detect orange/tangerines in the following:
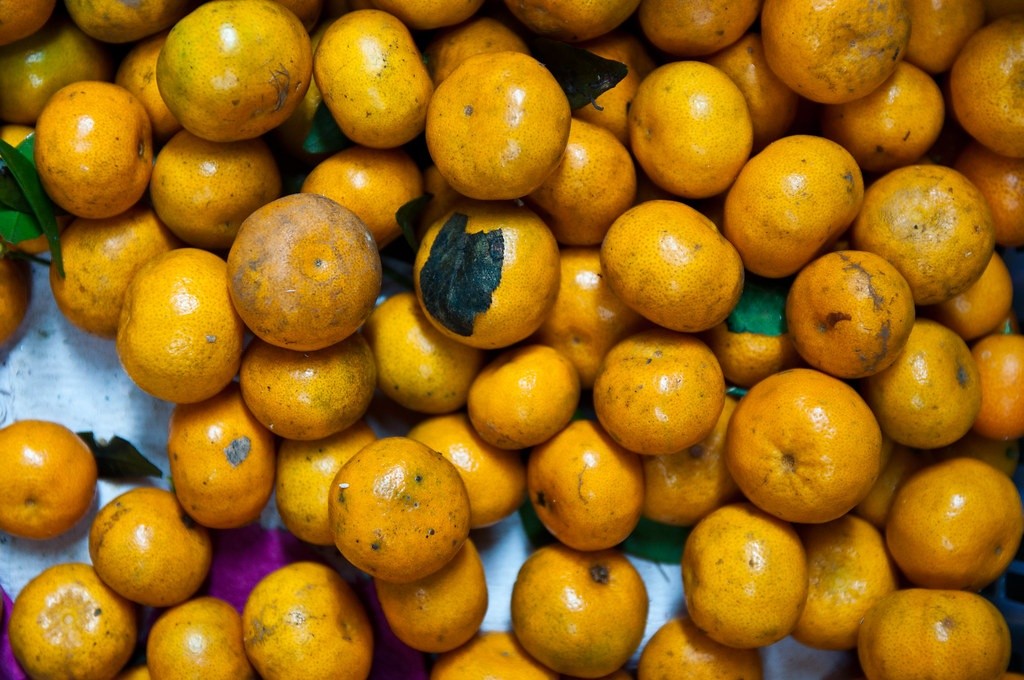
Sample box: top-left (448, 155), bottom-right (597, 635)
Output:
top-left (0, 0), bottom-right (1024, 680)
